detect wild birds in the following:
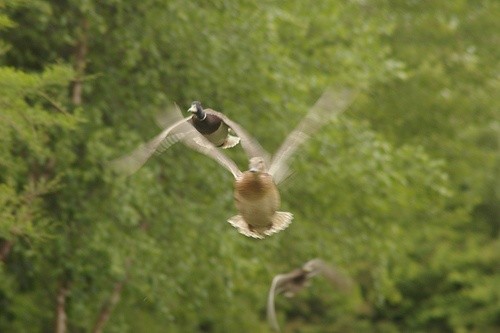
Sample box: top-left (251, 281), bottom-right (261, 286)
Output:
top-left (123, 99), bottom-right (288, 185)
top-left (148, 86), bottom-right (348, 239)
top-left (266, 255), bottom-right (352, 333)
